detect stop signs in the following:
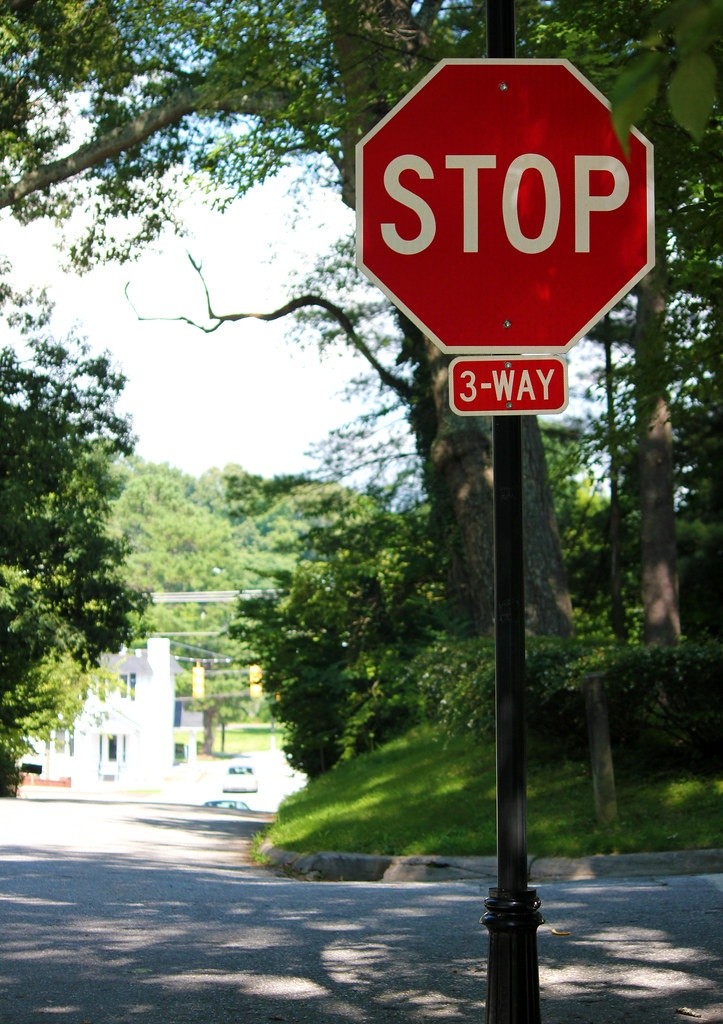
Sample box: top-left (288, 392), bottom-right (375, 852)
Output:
top-left (356, 57), bottom-right (656, 355)
